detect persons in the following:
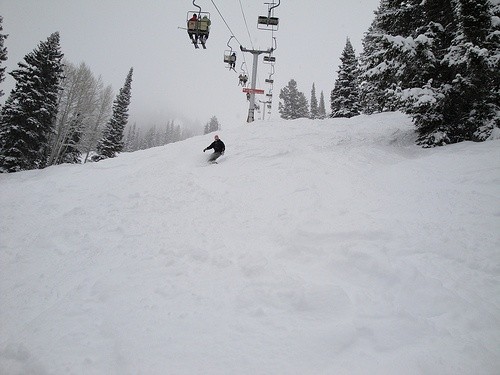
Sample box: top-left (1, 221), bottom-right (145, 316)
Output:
top-left (242, 75), bottom-right (248, 87)
top-left (230, 53), bottom-right (236, 69)
top-left (199, 15), bottom-right (211, 49)
top-left (187, 14), bottom-right (199, 48)
top-left (247, 93), bottom-right (250, 99)
top-left (238, 74), bottom-right (242, 85)
top-left (203, 135), bottom-right (226, 164)
